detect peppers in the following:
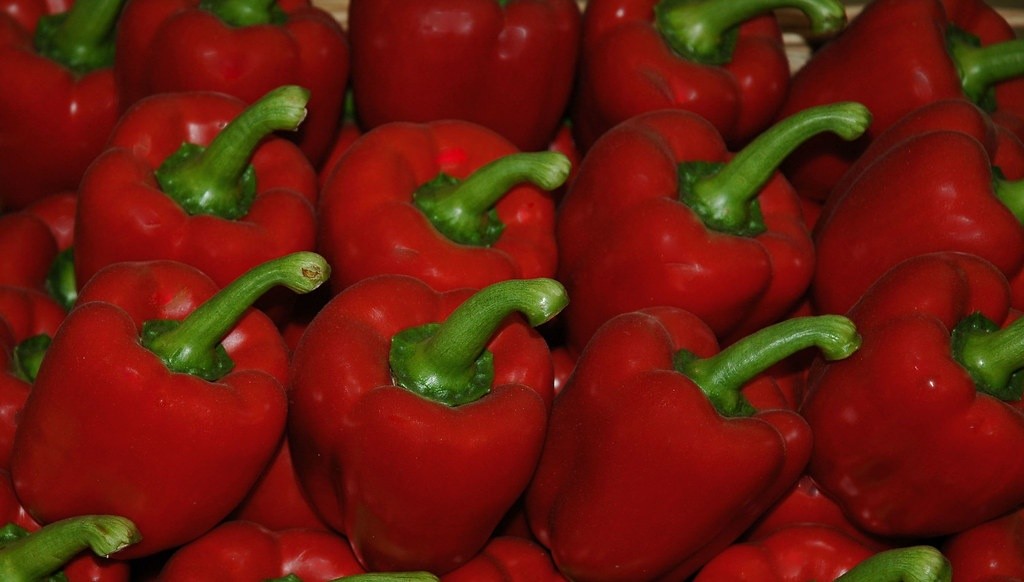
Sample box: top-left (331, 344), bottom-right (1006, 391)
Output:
top-left (0, 0), bottom-right (1024, 582)
